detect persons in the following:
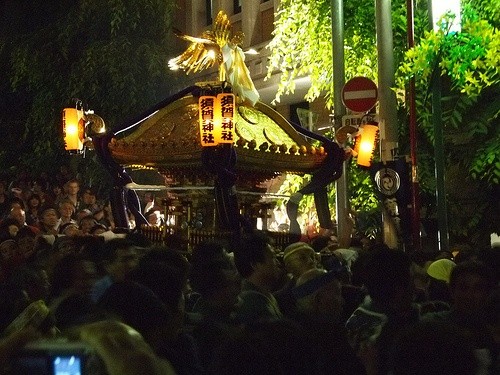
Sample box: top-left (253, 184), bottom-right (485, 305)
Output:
top-left (0, 165), bottom-right (500, 375)
